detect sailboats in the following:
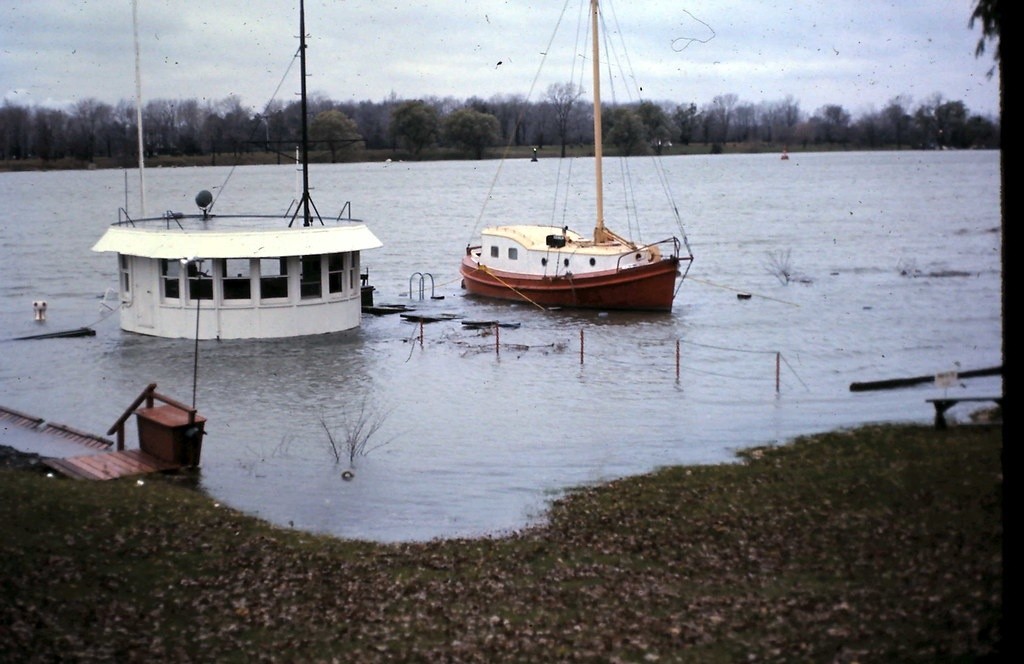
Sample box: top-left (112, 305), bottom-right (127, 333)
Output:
top-left (780, 144), bottom-right (790, 159)
top-left (461, 0), bottom-right (693, 313)
